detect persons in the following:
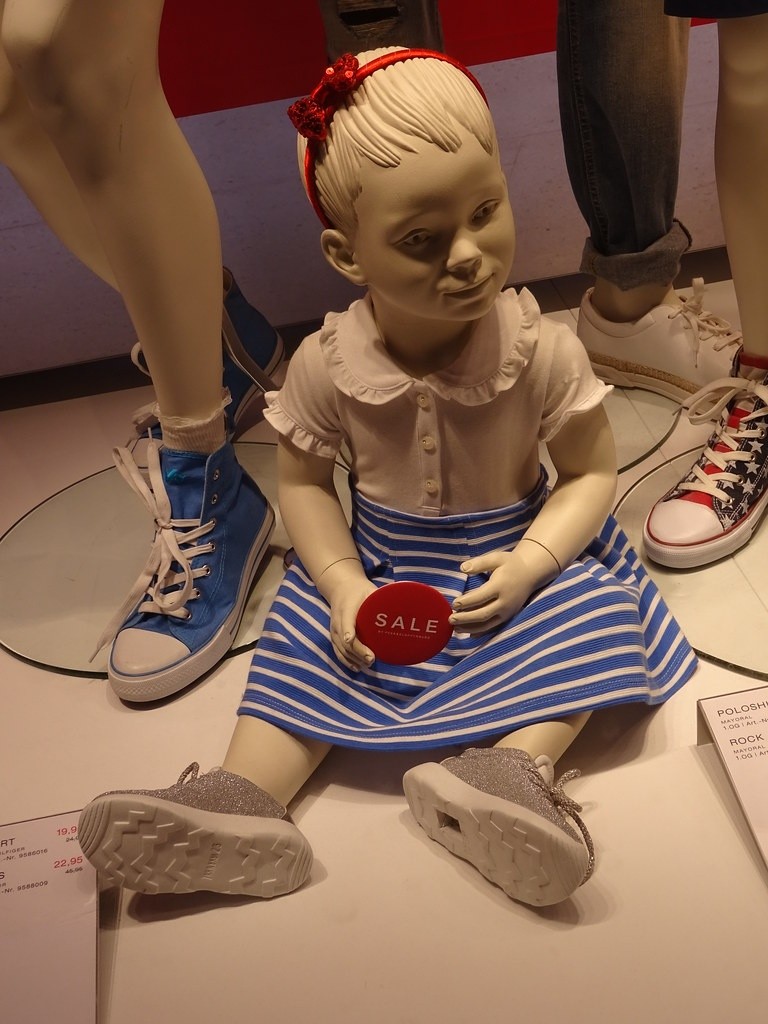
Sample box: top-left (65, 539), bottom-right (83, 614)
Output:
top-left (554, 1), bottom-right (768, 569)
top-left (0, 1), bottom-right (286, 706)
top-left (76, 43), bottom-right (699, 908)
